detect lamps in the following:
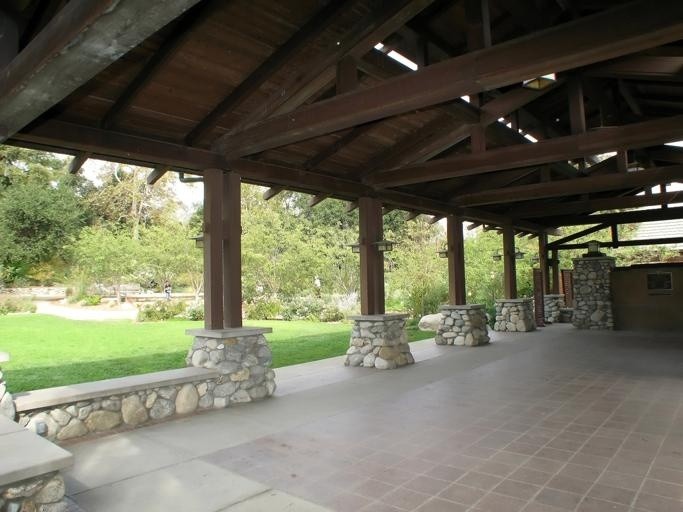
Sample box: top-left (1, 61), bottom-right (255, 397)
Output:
top-left (374, 239), bottom-right (399, 251)
top-left (522, 72), bottom-right (556, 90)
top-left (514, 247), bottom-right (527, 259)
top-left (435, 243), bottom-right (448, 258)
top-left (493, 247), bottom-right (504, 261)
top-left (346, 244), bottom-right (360, 253)
top-left (188, 233), bottom-right (203, 248)
